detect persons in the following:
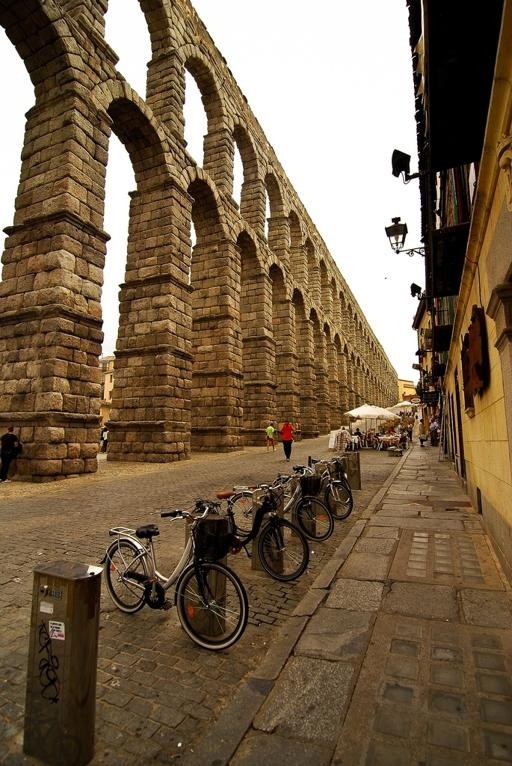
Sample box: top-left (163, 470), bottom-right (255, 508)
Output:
top-left (265, 420), bottom-right (296, 462)
top-left (0, 426), bottom-right (19, 483)
top-left (342, 400), bottom-right (419, 449)
top-left (419, 418), bottom-right (439, 447)
top-left (100, 428), bottom-right (109, 452)
